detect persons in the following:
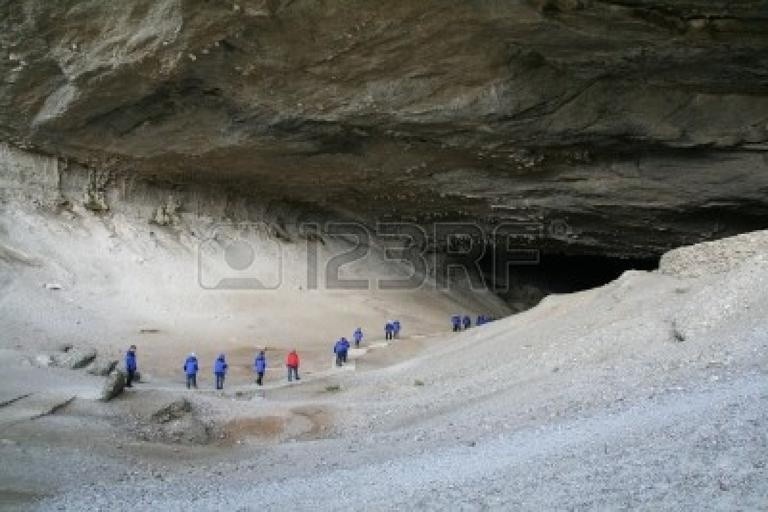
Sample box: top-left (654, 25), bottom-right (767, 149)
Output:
top-left (340, 337), bottom-right (350, 360)
top-left (392, 320), bottom-right (400, 337)
top-left (213, 353), bottom-right (228, 389)
top-left (450, 313), bottom-right (488, 332)
top-left (125, 344), bottom-right (138, 387)
top-left (353, 327), bottom-right (364, 349)
top-left (285, 348), bottom-right (300, 382)
top-left (384, 319), bottom-right (395, 340)
top-left (334, 337), bottom-right (345, 367)
top-left (183, 352), bottom-right (200, 390)
top-left (255, 351), bottom-right (266, 386)
